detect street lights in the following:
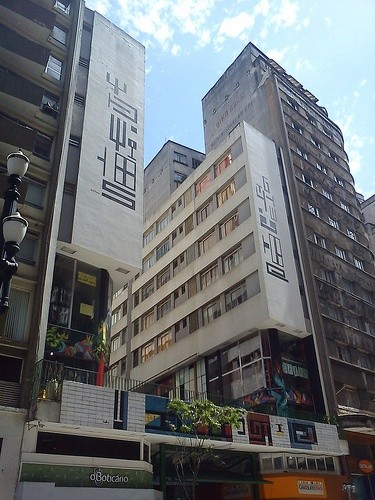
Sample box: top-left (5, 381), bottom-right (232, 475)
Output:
top-left (0, 146), bottom-right (32, 262)
top-left (0, 208), bottom-right (29, 318)
top-left (342, 484), bottom-right (356, 500)
